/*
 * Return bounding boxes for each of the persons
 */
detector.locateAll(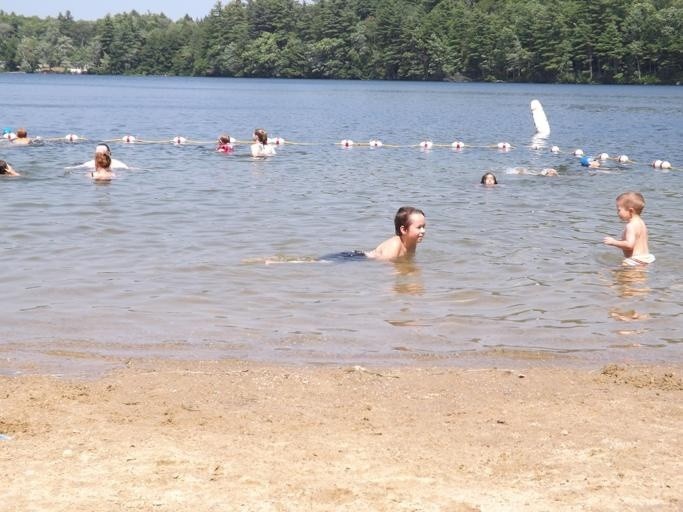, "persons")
[0,160,20,176]
[85,152,116,178]
[242,207,426,267]
[249,128,277,158]
[481,173,497,184]
[64,144,128,170]
[14,129,32,144]
[216,135,231,151]
[603,191,656,266]
